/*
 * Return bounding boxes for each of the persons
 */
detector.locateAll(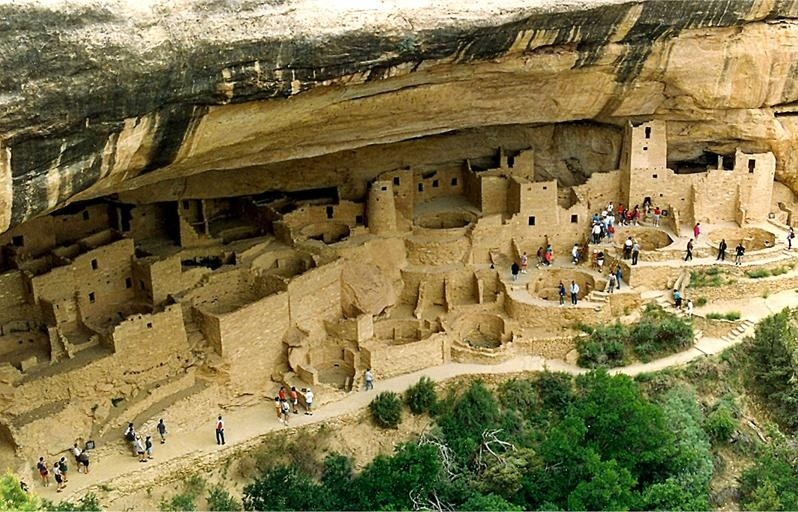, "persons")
[687,299,693,321]
[509,201,667,307]
[715,239,727,261]
[36,442,90,492]
[693,222,701,244]
[215,415,226,445]
[684,238,694,261]
[734,243,746,265]
[273,385,316,425]
[673,289,681,310]
[363,368,375,392]
[124,422,154,463]
[785,227,794,250]
[156,418,168,443]
